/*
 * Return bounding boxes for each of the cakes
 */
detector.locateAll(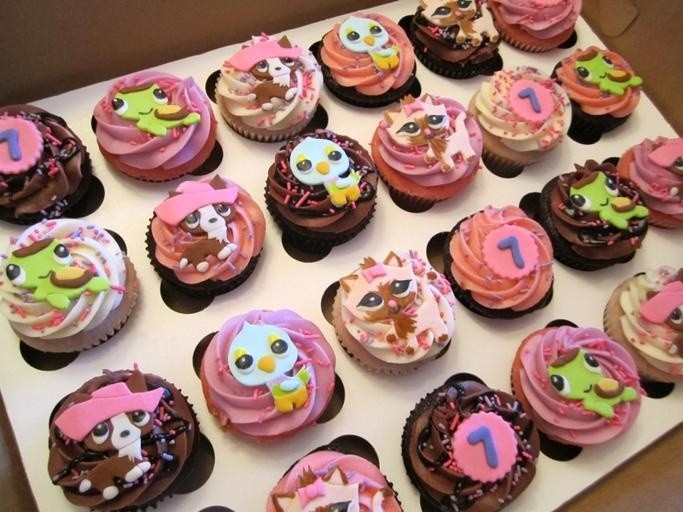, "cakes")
[601,264,683,400]
[425,204,555,320]
[508,319,646,463]
[399,371,541,511]
[204,31,328,144]
[1,215,140,372]
[397,0,503,80]
[143,173,268,315]
[320,247,459,377]
[467,65,573,180]
[484,1,584,54]
[368,91,484,213]
[263,127,381,265]
[307,11,423,109]
[191,307,346,443]
[46,360,216,512]
[0,102,105,226]
[600,134,683,233]
[551,45,644,145]
[89,68,224,185]
[517,159,651,274]
[264,433,407,512]
